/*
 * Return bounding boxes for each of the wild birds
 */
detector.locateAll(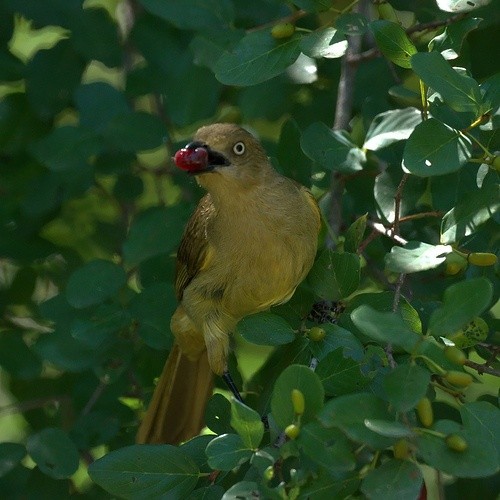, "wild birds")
[135,123,323,447]
